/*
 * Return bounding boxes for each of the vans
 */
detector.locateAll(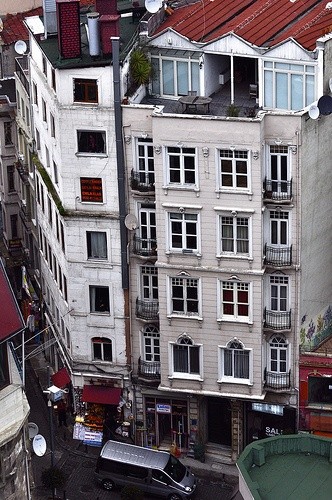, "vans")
[93,439,197,500]
